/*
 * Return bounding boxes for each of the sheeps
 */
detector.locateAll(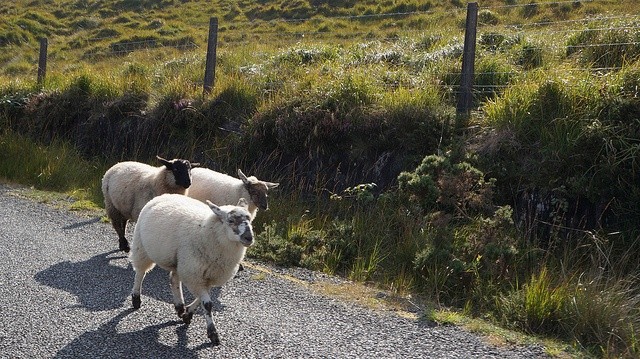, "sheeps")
[126,193,254,347]
[102,154,202,255]
[186,162,281,282]
